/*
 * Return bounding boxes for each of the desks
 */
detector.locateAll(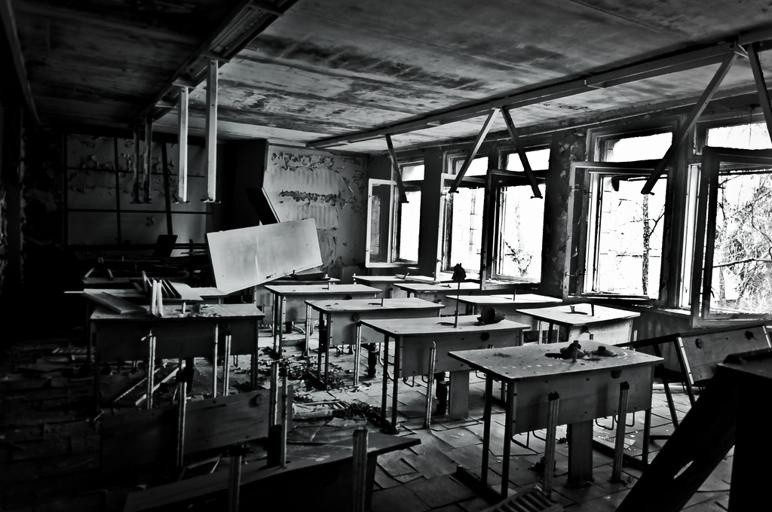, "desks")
[613,317,772,442]
[82,273,267,407]
[448,338,666,507]
[265,273,648,431]
